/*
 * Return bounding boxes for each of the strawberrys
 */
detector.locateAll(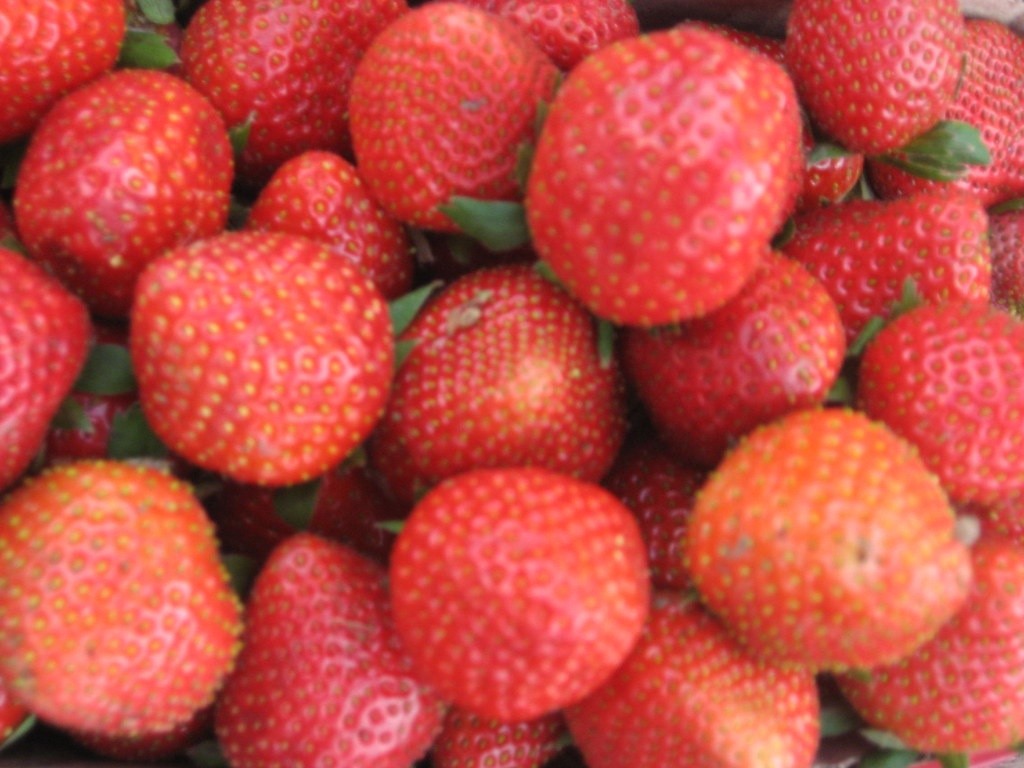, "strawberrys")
[0,0,1024,768]
